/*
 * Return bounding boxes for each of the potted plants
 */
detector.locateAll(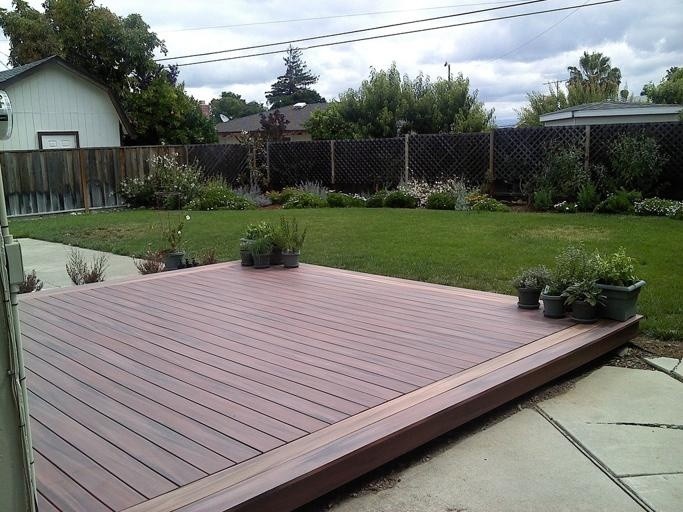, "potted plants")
[510,244,645,324]
[238,215,309,269]
[158,213,186,271]
